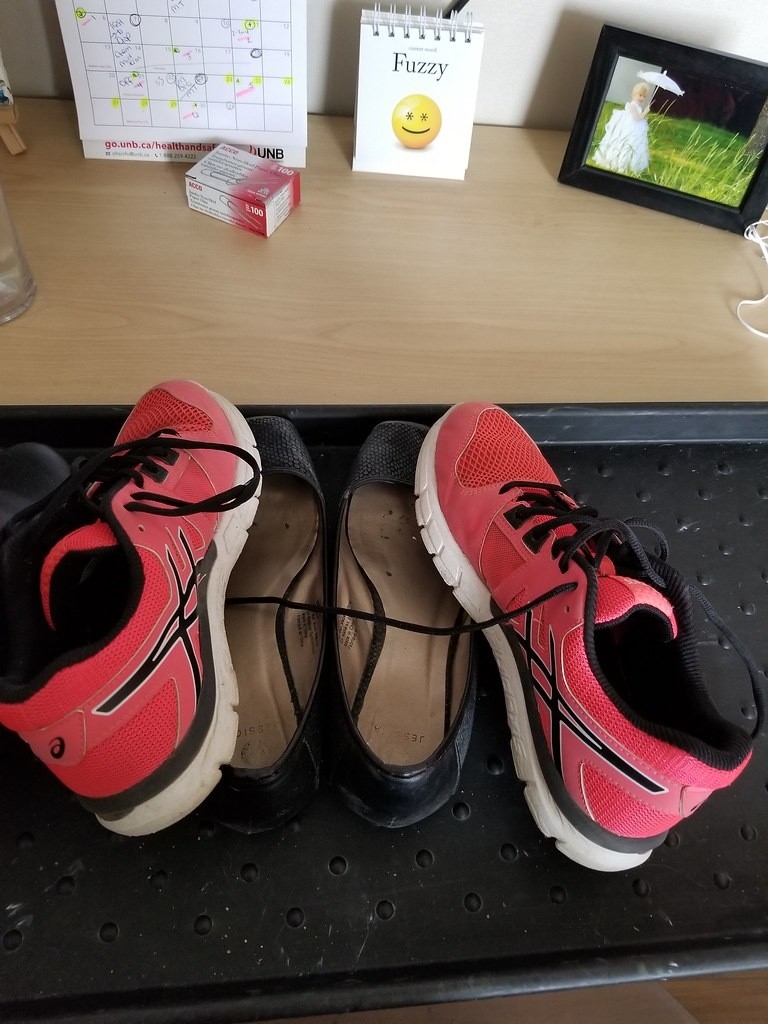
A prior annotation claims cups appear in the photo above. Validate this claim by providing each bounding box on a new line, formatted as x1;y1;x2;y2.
0;189;37;326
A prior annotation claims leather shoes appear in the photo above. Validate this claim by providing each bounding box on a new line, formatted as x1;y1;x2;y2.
198;414;482;830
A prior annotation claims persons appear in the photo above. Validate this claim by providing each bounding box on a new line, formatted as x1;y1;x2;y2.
592;83;650;173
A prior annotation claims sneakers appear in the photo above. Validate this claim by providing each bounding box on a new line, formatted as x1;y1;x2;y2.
2;377;262;837
414;399;766;873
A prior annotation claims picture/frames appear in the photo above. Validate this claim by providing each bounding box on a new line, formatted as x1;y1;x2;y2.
557;23;768;238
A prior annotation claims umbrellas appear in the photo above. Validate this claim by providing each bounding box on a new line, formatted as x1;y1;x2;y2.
637;70;685;104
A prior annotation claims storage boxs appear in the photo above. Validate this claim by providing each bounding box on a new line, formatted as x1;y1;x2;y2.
184;142;301;239
